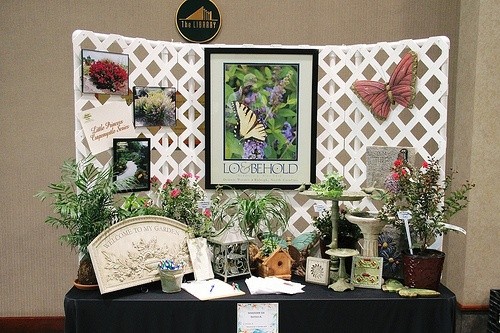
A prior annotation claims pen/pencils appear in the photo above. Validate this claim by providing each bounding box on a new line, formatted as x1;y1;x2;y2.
157;258;186;270
210;285;214;292
232;282;240;290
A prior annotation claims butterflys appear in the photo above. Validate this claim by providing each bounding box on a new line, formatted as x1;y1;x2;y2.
232;101;269;144
352;50;417;121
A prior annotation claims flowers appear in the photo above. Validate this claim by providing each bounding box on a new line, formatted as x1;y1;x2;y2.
373;156;476;254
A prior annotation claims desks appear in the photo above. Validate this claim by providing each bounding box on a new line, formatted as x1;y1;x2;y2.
63;269;457;333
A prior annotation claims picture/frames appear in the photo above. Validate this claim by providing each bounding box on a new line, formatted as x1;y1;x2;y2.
81;48;129;95
113;138;151;193
204;47;319;190
350;256;383;289
133;86;176;127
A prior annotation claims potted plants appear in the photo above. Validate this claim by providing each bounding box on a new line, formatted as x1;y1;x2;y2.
403;249;446;289
311;209;364;275
33;152;124;291
